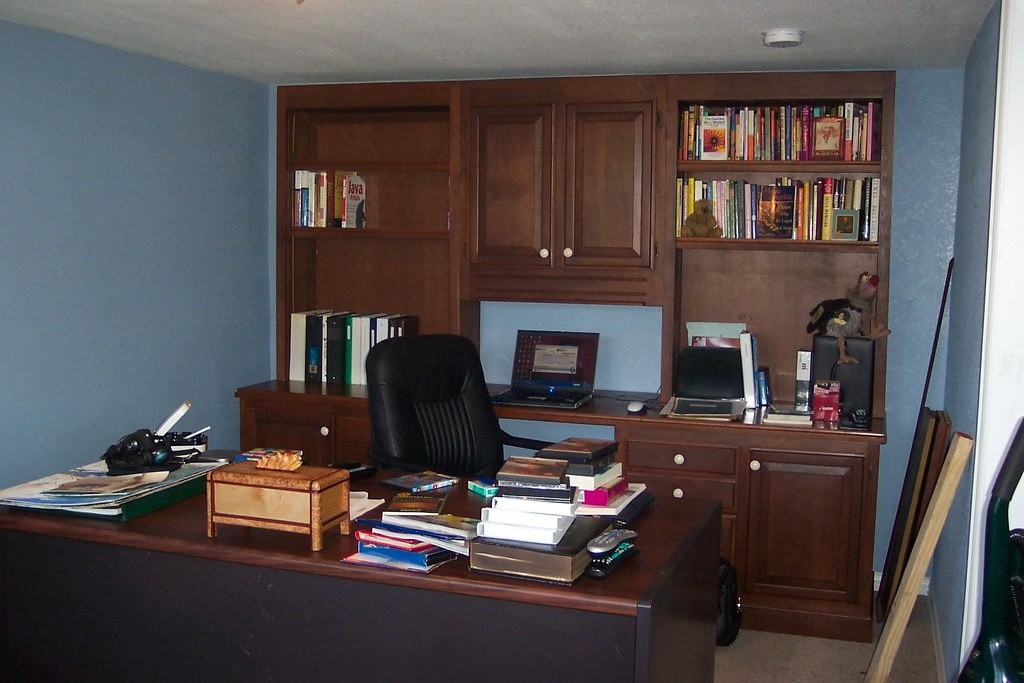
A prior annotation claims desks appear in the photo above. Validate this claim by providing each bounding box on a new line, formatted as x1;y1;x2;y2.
0;447;722;683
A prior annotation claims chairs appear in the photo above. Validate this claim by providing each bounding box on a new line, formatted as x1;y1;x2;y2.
364;334;555;477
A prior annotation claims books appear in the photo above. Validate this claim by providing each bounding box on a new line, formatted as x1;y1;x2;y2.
0;104;882;587
497;456;569;485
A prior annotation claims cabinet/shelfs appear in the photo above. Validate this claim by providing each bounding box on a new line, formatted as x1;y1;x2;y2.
232;70;896;643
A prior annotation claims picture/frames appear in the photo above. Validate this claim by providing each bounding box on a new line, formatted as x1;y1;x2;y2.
830;208;861;241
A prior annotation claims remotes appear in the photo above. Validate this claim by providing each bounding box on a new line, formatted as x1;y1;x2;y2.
585;540;640;578
586;528;638;557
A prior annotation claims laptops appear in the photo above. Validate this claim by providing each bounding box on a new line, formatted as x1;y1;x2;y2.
491;329;599;409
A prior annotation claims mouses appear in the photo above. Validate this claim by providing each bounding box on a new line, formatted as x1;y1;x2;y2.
627;401;648;415
